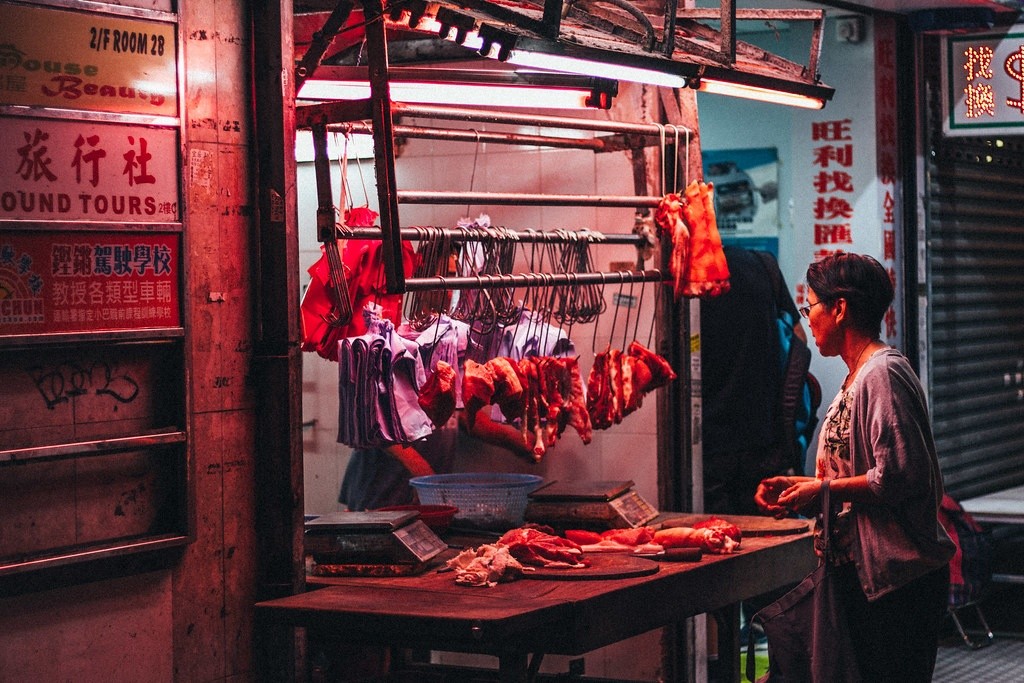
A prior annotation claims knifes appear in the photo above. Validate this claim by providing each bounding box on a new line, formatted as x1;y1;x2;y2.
585;548;702;560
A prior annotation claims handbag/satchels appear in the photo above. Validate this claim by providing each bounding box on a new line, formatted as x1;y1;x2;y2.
746;479;862;683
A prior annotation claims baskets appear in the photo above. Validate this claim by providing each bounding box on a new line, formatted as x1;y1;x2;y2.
409;472;542;532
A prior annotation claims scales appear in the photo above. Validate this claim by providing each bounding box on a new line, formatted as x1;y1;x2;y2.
524;479;661;531
302;510;450;567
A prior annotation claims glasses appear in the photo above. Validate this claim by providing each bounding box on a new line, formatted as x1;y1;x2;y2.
799;298;826;318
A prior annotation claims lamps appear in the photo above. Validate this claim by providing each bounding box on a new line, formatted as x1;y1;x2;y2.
384;1;836;110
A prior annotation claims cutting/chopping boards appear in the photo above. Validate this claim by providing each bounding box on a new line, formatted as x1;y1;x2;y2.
517;552;660;579
661;515;809;535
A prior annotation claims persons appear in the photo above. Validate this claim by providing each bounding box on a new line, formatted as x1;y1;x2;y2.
754;251;958;683
338;238;549;511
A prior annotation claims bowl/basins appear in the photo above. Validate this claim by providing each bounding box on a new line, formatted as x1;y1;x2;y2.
375;505;457;533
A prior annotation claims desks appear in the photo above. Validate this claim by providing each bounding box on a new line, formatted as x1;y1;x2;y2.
253;513;818;683
959;485;1024;641
